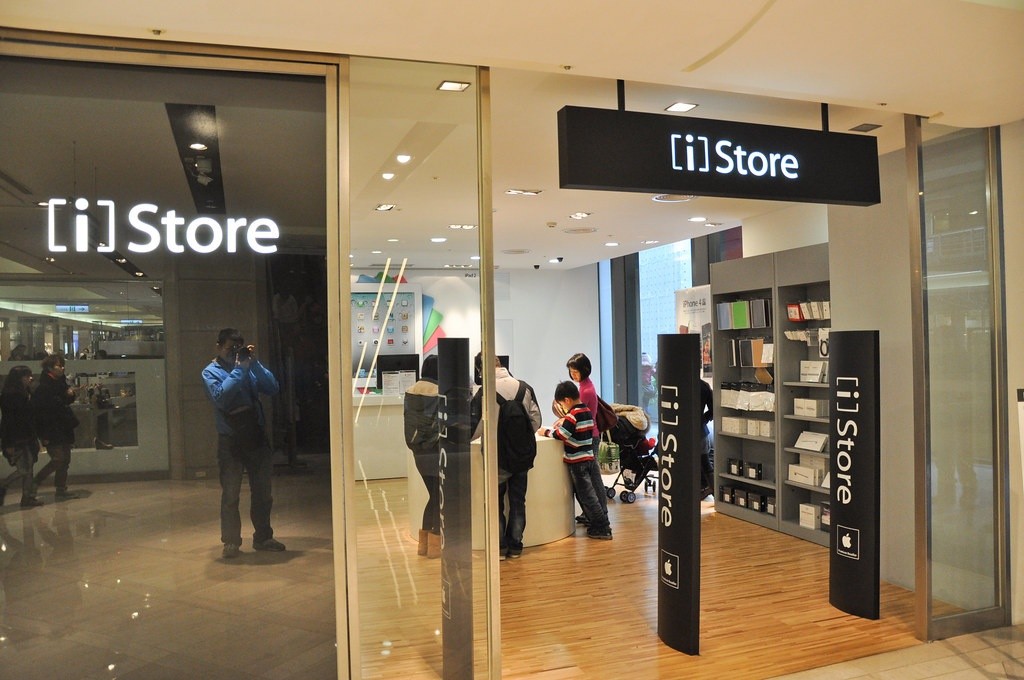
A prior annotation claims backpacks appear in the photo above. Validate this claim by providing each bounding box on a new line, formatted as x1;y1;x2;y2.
596;394;617;432
496;379;538;475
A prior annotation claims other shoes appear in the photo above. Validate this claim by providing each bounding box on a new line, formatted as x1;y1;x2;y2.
54;486;80;500
20;495;40;507
587;526;612;539
584;519;592;526
575;515;585;521
0;477;8;506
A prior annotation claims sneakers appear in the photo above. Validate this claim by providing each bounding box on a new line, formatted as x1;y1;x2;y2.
500;541;523;560
222;543;239;558
252;533;285;552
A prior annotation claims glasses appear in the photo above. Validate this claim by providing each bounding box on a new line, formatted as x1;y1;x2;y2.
56;364;64;367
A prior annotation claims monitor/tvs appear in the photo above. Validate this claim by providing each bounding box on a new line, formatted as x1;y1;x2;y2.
377;354;419;389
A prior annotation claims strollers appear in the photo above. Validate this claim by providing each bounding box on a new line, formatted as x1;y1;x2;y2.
604;404;660;506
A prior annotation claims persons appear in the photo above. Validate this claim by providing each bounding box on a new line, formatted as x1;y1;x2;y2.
403;354;442;559
0;342;107;508
201;328;286;559
553;353;608;526
469;354;542;561
538;381;613;539
639;433;655;455
700;379;716;497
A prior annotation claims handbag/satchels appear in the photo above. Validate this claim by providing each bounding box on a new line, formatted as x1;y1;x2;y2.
217;400;260;435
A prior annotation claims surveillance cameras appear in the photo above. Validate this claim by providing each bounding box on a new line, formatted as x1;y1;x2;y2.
534;265;539;269
557;258;563;262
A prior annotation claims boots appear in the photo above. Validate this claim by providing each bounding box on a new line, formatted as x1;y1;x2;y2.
427;531;441;559
417;528;432;555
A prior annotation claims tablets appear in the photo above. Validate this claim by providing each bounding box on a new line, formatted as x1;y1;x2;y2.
351;282;423;388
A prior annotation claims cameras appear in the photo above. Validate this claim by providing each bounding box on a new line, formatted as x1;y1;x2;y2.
230;345;250;362
